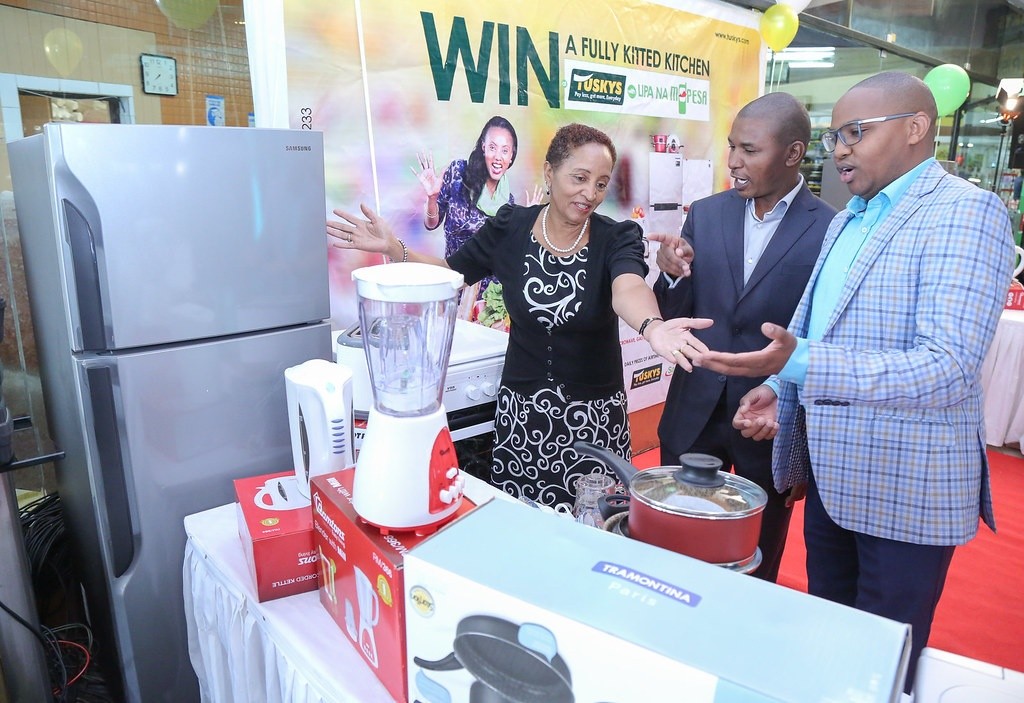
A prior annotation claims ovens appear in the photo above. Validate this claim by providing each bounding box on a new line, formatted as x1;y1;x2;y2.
445;401;498;482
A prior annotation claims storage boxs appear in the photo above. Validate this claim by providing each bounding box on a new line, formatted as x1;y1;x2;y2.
310;468;477;703
233;471;319;604
1004;283;1024;310
403;496;912;703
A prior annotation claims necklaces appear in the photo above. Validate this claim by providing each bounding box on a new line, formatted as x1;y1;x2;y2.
542;203;588;253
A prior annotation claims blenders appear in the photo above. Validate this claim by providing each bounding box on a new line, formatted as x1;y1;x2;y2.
352;262;466;536
354;565;378;669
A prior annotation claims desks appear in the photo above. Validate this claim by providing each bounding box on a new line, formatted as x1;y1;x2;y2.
183;469;531;703
980;309;1024;456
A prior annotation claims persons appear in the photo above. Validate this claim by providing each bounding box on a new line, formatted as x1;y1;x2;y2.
411;116;544;309
328;123;714;513
646;91;839;584
699;72;1016;696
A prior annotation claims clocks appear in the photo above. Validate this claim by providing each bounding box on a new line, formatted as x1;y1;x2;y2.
140;53;178;96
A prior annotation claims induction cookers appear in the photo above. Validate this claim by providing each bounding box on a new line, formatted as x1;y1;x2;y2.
332;318;509;417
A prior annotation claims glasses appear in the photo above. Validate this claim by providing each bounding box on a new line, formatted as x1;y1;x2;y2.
821;114;920;153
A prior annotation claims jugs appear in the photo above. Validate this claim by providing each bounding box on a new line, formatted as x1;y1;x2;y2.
570;476;627;533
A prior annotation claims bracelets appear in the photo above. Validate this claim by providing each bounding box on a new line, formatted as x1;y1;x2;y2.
426;203;439;218
638;318;664;336
389;237;407;263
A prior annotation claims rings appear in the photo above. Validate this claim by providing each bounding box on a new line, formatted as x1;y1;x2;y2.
681;346;684;353
672;350;679;356
348;234;352;242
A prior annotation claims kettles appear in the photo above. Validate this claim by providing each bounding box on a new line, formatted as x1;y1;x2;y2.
284;358;357;499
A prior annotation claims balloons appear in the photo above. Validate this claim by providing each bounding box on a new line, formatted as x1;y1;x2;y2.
760;3;799;52
923;64;970;117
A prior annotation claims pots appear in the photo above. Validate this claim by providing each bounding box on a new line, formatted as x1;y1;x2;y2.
412;615;577;703
650;133;684;154
573;441;768;564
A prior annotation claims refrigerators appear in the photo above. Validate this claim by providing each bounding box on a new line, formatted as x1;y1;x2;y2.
648;151;684;240
5;122;331;702
